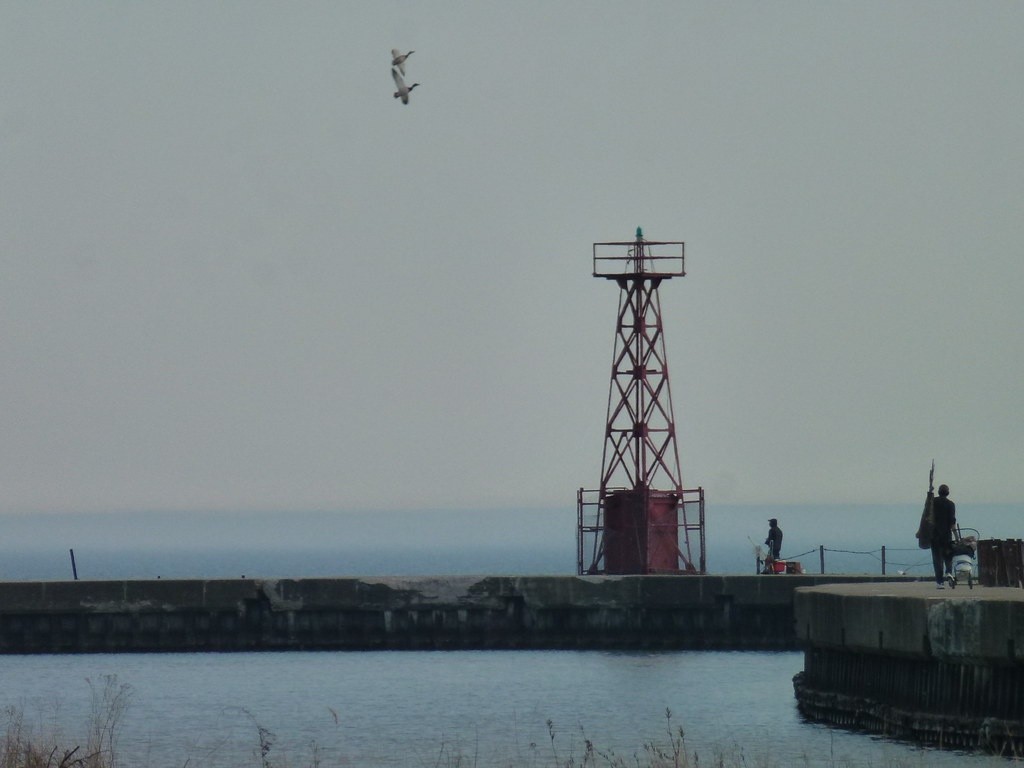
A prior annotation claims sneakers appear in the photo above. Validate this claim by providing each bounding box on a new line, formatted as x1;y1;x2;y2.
945;571;956;586
937;583;945;589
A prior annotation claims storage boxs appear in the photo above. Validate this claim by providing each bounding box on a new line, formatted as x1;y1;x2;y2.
786;561;801;574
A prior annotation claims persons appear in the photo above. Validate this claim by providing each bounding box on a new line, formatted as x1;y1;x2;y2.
760;518;783;575
915;484;961;589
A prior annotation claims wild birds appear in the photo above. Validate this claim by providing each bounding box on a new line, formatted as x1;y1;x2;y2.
386;46;416;77
386;66;421;106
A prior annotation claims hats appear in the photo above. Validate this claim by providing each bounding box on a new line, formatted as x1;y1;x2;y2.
768;518;777;522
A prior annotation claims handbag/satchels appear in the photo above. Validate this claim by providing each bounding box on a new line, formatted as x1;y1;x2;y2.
918;492;936;549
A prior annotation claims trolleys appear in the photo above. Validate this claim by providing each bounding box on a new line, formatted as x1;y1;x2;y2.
939;524;981;589
